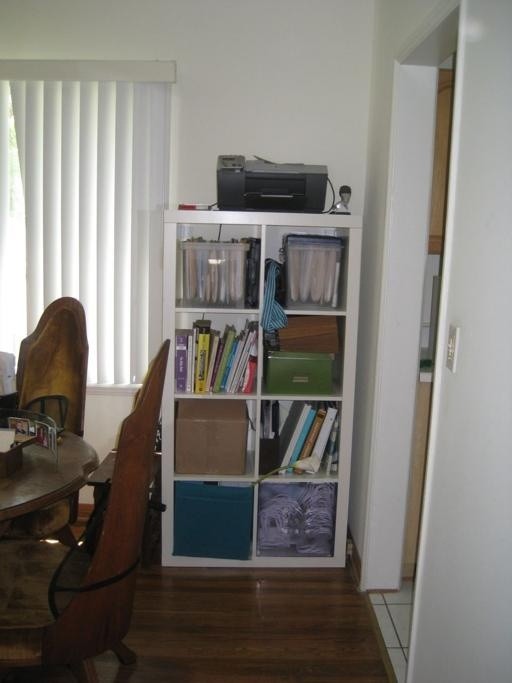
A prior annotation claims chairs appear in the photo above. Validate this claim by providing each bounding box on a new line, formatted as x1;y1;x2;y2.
2;339;170;682
16;298;91;536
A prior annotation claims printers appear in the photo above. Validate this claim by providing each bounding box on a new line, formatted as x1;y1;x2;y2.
217;154;328;214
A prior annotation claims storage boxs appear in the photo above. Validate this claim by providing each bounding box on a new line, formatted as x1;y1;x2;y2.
264;351;337;394
282;234;342;312
184;241;249;310
170;479;255;561
174;398;256;476
276;315;340;353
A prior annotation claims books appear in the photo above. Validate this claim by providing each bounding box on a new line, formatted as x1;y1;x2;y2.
283;231;341;310
271;400;341;476
174;232;259;397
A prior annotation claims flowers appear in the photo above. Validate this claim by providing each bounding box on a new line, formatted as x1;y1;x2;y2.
254;452;320;484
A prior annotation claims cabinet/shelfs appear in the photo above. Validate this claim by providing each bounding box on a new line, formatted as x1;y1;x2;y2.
159;205;364;568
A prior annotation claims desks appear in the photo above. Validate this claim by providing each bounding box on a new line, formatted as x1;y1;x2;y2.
3;426;94;538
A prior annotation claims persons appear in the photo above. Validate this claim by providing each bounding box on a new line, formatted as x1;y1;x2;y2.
15;421;26;434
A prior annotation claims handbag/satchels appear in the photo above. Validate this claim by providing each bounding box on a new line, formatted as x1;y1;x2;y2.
265;258;285;306
